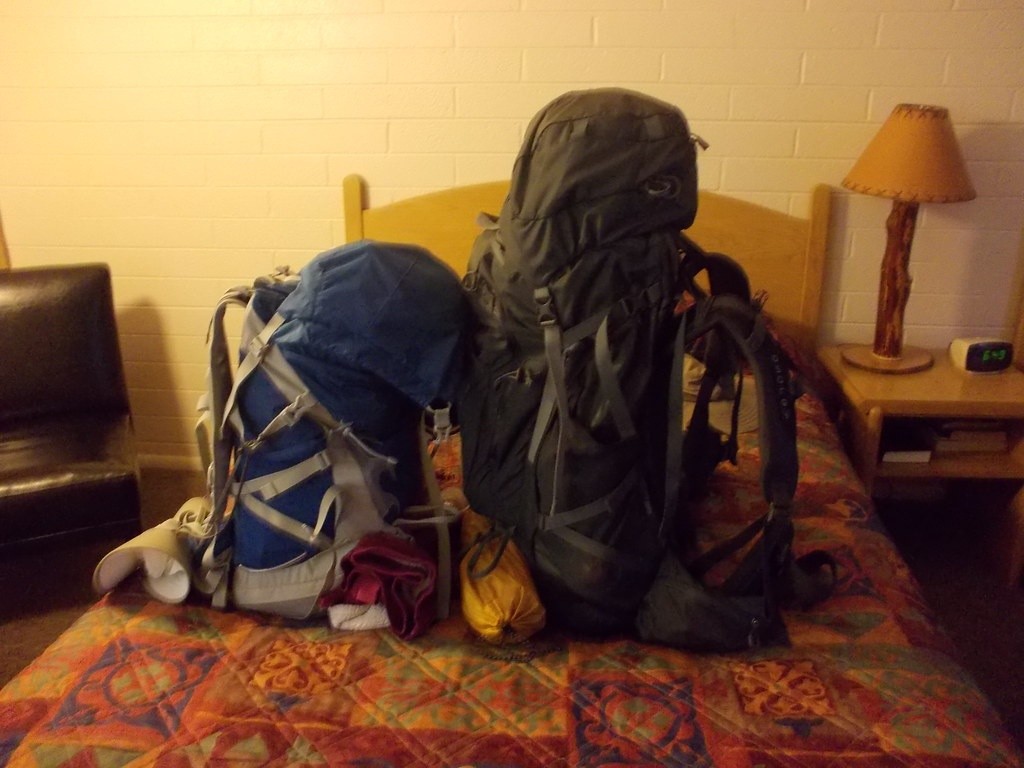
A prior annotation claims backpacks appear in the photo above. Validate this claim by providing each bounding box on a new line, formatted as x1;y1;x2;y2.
194;239;461;632
460;86;837;650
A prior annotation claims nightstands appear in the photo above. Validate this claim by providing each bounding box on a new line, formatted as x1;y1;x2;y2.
814;341;1024;596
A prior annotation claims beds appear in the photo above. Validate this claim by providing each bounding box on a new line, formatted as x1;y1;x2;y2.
0;87;1024;768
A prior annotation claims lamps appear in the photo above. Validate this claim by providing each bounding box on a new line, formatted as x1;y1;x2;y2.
840;102;979;375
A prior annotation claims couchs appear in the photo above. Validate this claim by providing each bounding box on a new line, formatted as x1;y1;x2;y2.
0;263;148;623
